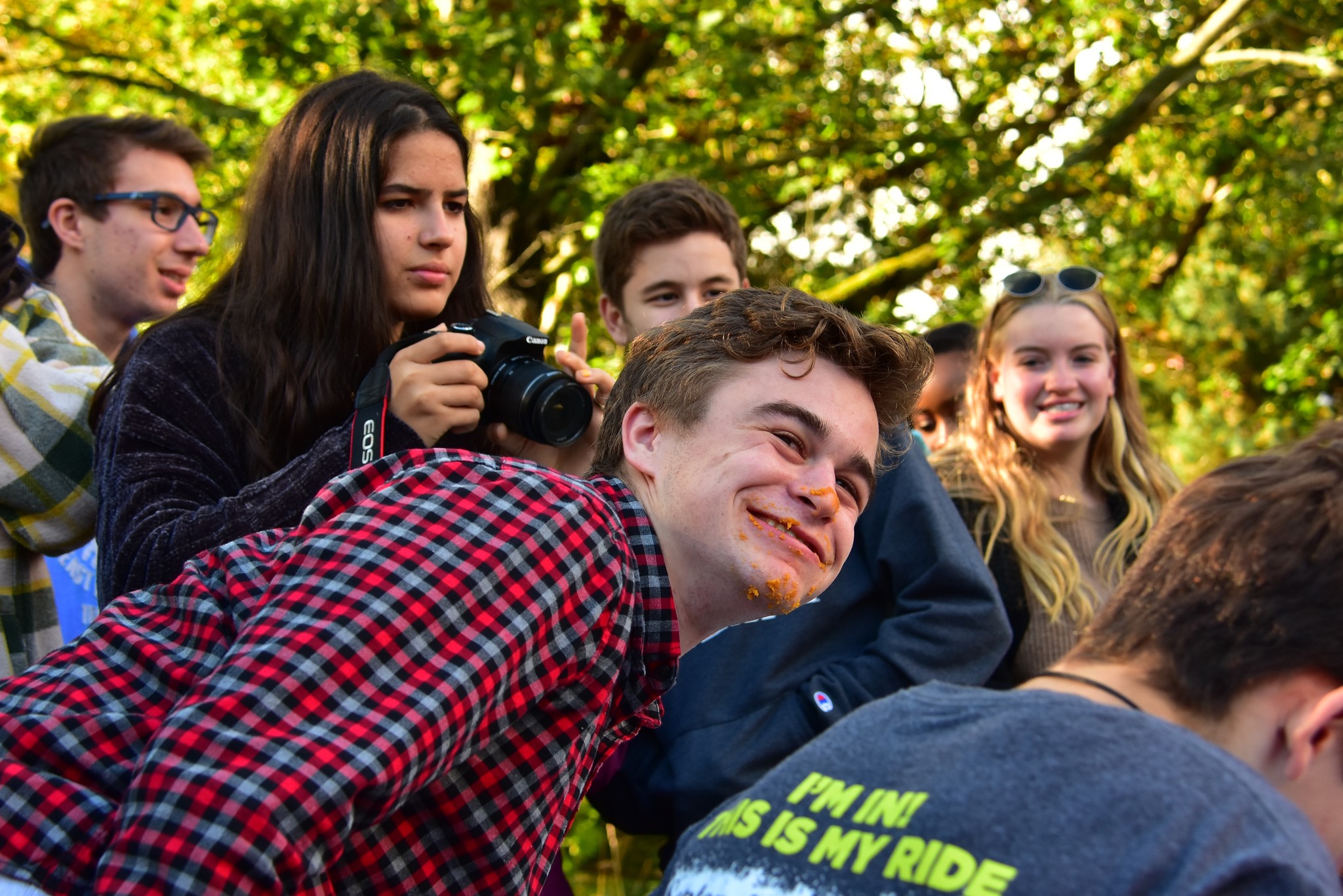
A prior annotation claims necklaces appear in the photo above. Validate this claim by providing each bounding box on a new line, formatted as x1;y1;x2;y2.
1037;670;1140;709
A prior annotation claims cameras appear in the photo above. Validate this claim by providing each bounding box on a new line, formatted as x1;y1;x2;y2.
430;309;591;449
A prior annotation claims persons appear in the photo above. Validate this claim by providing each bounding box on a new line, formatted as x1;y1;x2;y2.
0;284;930;896
662;408;1343;896
0;113;219;687
910;316;988;454
926;266;1183;692
99;69;610;618
538;175;1016;835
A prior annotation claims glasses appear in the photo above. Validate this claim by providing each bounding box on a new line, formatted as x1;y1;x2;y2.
40;191;218;248
991;265;1104;326
